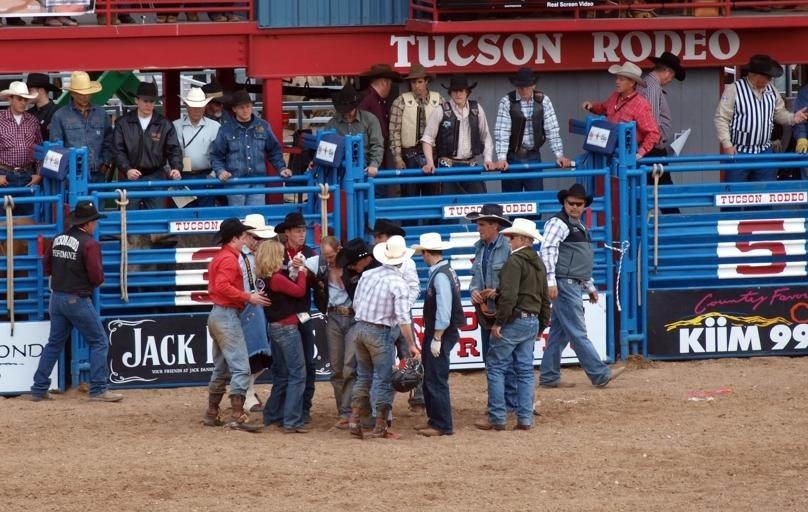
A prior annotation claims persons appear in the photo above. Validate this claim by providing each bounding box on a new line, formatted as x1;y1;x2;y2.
636;52;686;213
466;202;548;429
582;61;661;160
30;201;124;403
540;183;626;387
1;0;240;27
793;84;808;180
207;210;466;439
0;65;569;320
713;55;808;211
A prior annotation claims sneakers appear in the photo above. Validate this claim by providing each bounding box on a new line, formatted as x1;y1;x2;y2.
0;12;241;26
30;393;54;401
89;391;123;402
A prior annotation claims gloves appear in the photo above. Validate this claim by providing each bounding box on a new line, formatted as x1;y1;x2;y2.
431;337;441;358
795;138;808;153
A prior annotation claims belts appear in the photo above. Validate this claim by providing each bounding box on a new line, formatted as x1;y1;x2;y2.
0;163;34;176
451;157;474;163
358;320;391;329
328;305;354;316
517;311;536;316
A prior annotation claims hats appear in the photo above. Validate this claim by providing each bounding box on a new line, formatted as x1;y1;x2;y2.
68;201;107;225
